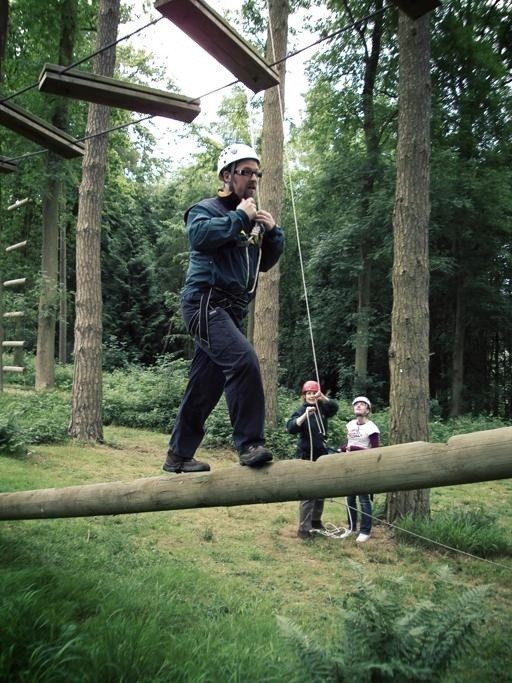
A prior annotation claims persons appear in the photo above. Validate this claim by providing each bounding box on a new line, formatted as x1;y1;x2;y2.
159;142;286;475
339;396;380;543
286;380;339;541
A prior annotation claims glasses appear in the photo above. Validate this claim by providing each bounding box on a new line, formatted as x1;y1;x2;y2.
233;169;263;178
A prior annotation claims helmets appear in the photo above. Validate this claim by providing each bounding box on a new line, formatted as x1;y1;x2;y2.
217;143;260;182
302;380;319;393
352;396;371;408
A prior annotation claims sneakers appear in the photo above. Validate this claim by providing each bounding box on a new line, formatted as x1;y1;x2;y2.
239;445;273;466
297;529;315;540
340;528;357;539
312;520;326;532
355;532;371;544
163;449;210;473
